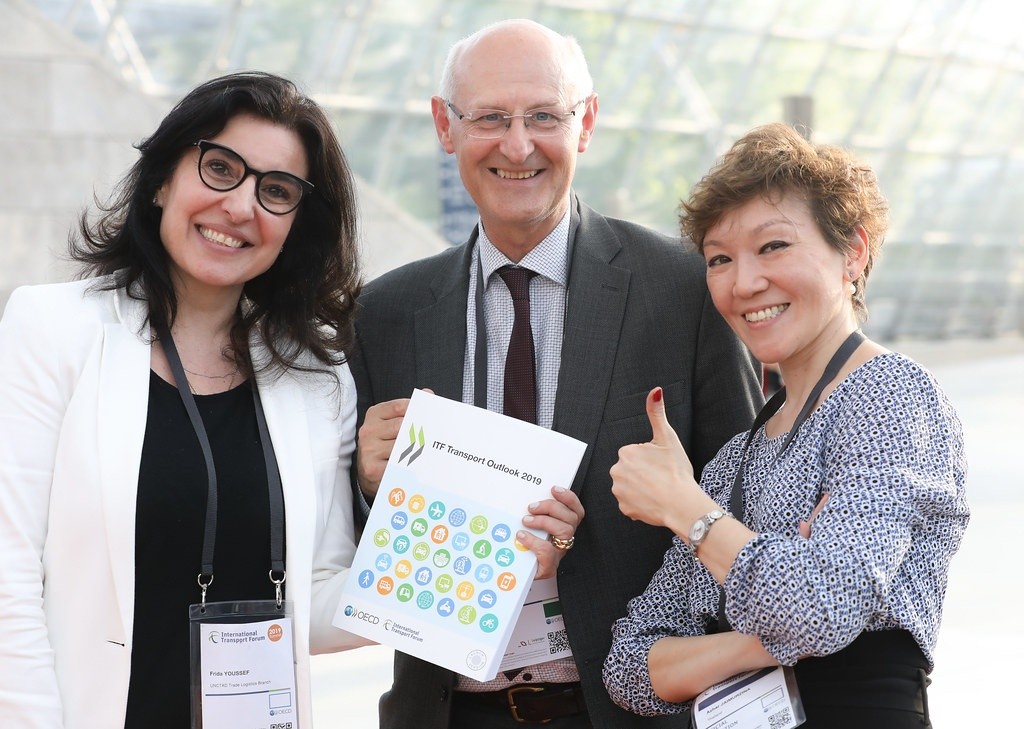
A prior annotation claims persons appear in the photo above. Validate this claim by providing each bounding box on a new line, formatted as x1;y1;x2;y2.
335;19;768;729
2;71;587;729
599;122;970;729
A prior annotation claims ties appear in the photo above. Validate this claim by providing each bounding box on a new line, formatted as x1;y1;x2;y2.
493;265;541;682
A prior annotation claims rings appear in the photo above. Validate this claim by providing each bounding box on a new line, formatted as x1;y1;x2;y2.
551;534;575;551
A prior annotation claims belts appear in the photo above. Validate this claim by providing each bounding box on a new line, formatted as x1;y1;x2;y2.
451;683;587;724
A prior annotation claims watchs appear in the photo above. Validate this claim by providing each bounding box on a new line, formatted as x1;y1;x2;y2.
689;510;735;557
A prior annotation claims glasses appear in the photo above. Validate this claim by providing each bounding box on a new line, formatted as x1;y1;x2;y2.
446;97;587;140
192;138;315;215
